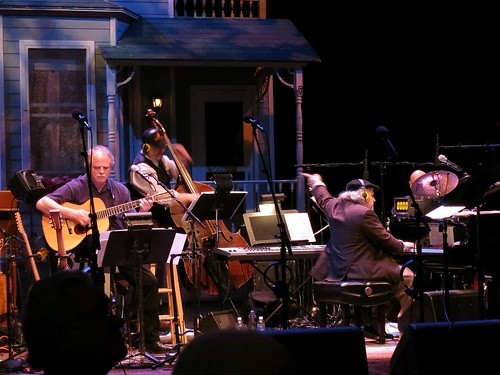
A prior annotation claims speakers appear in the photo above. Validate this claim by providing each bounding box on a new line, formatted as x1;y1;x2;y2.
397;289;481;332
388;319;500;375
171;325;369;375
195;309;239;336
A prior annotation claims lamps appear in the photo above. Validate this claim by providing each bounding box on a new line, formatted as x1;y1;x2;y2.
152;94;164;113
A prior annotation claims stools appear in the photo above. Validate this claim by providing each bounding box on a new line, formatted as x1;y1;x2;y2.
314;280;394;344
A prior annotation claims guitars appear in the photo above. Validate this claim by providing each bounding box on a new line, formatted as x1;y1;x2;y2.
47;209;74;272
41;189;176;253
14;210;41;281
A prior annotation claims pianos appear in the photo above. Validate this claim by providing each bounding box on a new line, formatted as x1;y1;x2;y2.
390;209;500;341
217;243;327;327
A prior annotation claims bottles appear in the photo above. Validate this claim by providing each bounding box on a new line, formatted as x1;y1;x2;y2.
236;317;244;331
248;310;256;330
257;316;266;331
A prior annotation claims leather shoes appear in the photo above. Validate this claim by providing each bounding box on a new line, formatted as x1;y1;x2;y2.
144;340;175;353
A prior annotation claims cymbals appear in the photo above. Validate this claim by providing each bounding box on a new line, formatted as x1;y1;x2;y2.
483;181;500;197
411;169;459;199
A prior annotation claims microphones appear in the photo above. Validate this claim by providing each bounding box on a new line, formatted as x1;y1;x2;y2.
481;182;500;198
240;114;266;132
438;155;472;180
376;126;395;149
72;108;92;130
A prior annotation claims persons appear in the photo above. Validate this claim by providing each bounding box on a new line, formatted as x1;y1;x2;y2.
36;146;175;354
128;127;201;233
391;170;438;241
300;173;415;320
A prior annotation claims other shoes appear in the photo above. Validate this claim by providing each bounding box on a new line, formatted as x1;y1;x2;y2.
363;326;393;341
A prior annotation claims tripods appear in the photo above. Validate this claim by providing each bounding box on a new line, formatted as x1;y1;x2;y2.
102;230;177;368
152;253;189;369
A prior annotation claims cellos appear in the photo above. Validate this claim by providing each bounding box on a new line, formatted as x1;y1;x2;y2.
144;107;255;319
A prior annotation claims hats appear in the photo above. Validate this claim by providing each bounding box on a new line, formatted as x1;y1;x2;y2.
346;179;379;191
143;128;168;149
